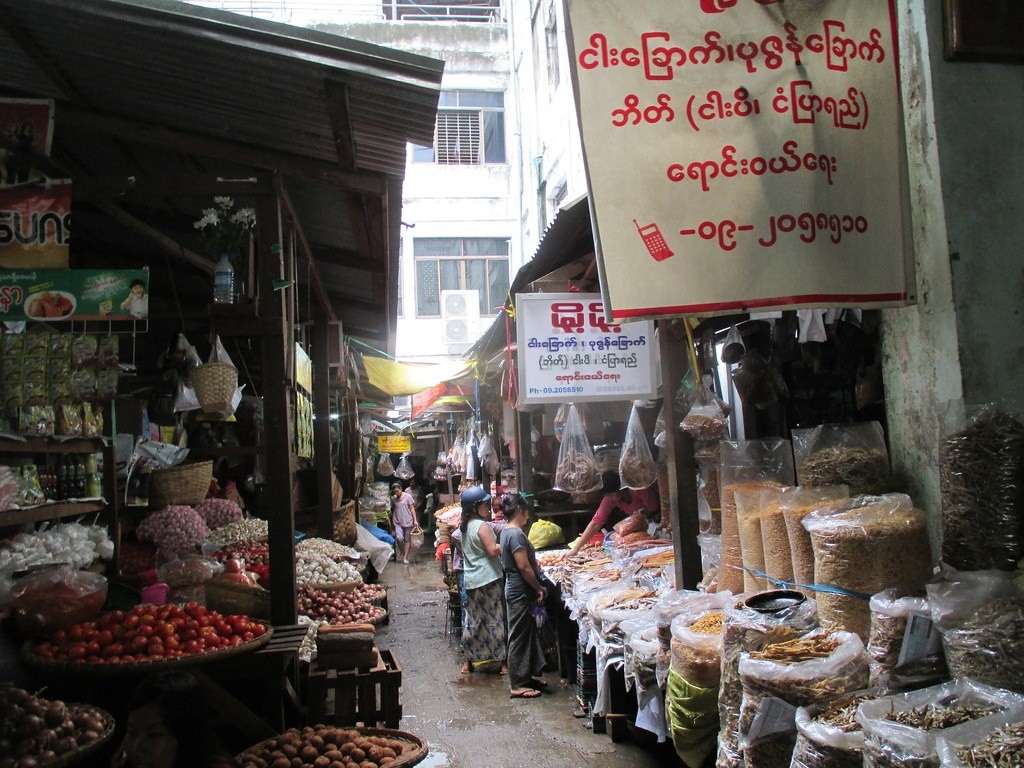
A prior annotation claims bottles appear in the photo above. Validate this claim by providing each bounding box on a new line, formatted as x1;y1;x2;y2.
39;453;101;500
213;253;234;305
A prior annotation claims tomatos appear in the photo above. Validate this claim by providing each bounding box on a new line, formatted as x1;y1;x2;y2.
30;601;266;664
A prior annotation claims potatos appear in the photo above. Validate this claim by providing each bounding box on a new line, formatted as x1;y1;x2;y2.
241;724;402;768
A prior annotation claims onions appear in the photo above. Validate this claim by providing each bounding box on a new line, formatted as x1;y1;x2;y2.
0;683;106;768
296;581;383;625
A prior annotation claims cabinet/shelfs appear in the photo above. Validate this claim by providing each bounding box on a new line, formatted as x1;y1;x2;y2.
445;600;461;648
0;436;121;627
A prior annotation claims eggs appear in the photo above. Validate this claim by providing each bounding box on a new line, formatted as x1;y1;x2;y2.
539;554;566;567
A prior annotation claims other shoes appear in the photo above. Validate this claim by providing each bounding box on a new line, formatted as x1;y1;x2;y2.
398;554;403;560
404;560;409;564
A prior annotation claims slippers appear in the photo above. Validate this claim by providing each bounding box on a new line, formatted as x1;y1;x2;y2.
462;664;475;673
510;688;541;698
500;668;509;674
522;679;547;687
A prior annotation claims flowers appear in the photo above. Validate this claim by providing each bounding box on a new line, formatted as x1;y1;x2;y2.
192;196;256;252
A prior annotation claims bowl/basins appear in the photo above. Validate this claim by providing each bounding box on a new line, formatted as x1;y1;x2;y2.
743;589;807;615
24;290;75;320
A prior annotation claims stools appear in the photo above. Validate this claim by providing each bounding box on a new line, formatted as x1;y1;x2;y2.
369;582;389;625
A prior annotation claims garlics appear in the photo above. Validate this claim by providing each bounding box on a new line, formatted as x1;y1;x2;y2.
295;549;359;585
135;498;244;559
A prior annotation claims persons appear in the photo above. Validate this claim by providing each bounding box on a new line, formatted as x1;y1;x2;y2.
405;478;424;526
458;487;508;674
451;522;510;645
498;493;550;698
391;481;418;564
562;470;661;557
120;279;148;319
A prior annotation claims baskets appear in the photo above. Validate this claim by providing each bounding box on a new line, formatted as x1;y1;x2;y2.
139;460;212;510
190;365;238;414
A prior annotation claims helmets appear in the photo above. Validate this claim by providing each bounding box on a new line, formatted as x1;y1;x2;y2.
461;487;491;506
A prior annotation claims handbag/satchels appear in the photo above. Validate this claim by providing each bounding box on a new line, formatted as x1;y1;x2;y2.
410;525;424;548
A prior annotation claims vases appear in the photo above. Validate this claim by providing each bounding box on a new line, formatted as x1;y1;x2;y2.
213;253;234;304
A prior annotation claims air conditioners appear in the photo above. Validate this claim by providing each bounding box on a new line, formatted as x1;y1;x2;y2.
439;289;480;345
